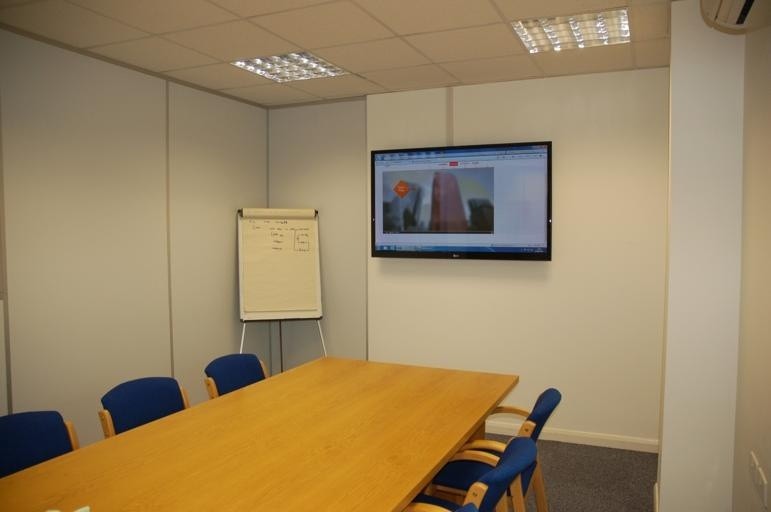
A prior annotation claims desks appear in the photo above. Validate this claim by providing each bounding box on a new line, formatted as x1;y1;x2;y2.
0;355;520;512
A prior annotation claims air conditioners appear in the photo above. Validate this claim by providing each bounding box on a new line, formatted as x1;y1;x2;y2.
699;0;771;35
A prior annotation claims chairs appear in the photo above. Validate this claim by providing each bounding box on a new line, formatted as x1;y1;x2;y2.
0;409;79;479
461;387;561;512
202;353;269;400
401;503;479;512
96;377;190;439
424;437;537;512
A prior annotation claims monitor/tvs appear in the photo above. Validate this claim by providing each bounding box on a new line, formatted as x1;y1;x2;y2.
370;140;553;262
